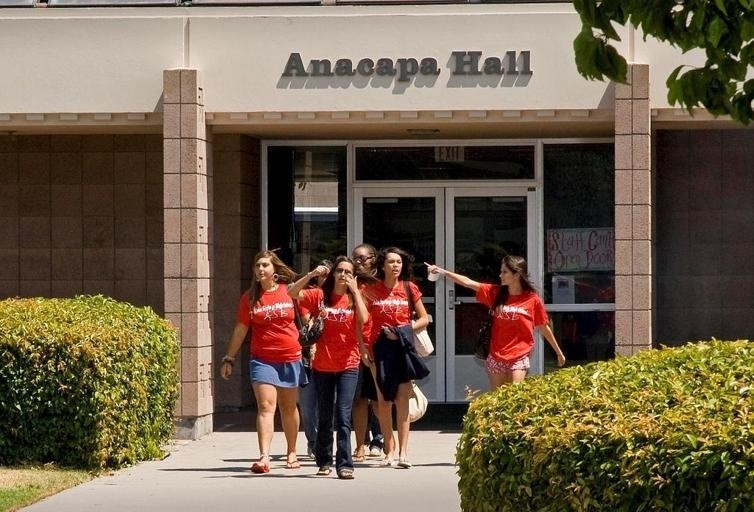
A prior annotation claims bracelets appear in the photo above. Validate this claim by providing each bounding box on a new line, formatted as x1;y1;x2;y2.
223;356;235;367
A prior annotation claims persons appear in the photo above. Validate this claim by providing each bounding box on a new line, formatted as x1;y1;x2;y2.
221;252;317;472
287;244;430;478
551;268;615;360
423;253;567;393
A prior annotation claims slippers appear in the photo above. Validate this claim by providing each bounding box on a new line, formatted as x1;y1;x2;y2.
308;447;332;475
251;462;269;473
337;469;353;479
352;443;381;462
287;461;300;468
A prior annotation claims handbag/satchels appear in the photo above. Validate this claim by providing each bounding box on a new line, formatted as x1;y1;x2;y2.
412;311;435;358
298;317;324;360
409;379;428;422
473;315;491;359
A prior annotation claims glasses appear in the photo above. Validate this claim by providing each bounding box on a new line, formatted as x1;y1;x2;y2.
351;255;373;263
335;268;351;274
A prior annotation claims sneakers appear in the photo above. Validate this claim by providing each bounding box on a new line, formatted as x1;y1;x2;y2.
398;452;412;467
380;454;393;467
